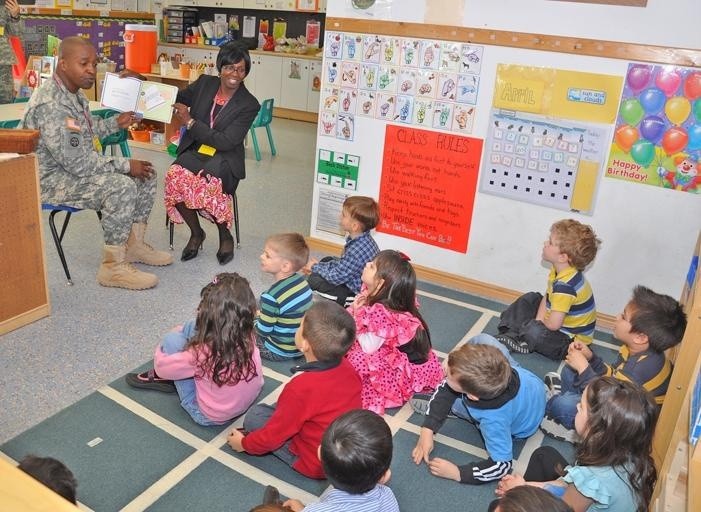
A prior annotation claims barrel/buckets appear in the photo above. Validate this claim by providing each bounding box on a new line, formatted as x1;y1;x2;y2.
122;23;159;74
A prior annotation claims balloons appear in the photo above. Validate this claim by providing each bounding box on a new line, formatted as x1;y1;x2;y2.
614;65;701;168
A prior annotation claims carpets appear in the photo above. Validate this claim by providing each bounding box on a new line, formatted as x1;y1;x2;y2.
1;278;625;512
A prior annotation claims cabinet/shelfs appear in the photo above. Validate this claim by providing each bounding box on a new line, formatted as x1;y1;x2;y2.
647;233;699;512
156;1;327;123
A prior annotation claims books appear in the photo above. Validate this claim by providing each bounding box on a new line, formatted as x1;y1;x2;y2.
14;34;64;101
98;71;179;125
187;20;231;39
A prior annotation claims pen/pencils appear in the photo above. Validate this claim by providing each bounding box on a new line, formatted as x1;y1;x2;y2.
162;54;170;62
187;62;206;71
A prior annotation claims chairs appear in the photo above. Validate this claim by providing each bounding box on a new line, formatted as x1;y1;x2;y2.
0;109;130;159
41;202;103;286
166;189;240;249
242;99;276;161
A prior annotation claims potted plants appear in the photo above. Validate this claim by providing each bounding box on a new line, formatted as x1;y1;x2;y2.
149;128;166;145
125;121;154;141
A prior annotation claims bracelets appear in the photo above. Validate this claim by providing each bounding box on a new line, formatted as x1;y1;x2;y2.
186;116;195;132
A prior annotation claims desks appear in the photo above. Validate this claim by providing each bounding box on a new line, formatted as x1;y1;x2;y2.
0;151;51;335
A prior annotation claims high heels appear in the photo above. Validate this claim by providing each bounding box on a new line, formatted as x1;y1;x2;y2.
216;235;234;265
181;229;206;261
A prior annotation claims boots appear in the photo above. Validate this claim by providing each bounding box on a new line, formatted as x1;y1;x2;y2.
96;222;174;290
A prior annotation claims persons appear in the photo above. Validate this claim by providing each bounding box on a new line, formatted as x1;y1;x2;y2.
15;34;175;289
299;195;381;311
125;271;266;428
227;298;365;481
411;332;551;486
492;218;604;366
164;40;261;265
17;453;78;508
290;248;446;417
250;409;403;512
487;376;658;512
0;0;23;103
538;285;688;448
250;230;317;364
493;486;575;512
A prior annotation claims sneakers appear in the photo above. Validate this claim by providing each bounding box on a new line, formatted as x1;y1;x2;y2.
409;395;458;419
539;411;584;446
493;332;529;354
125;368;175;393
543;372;562;402
